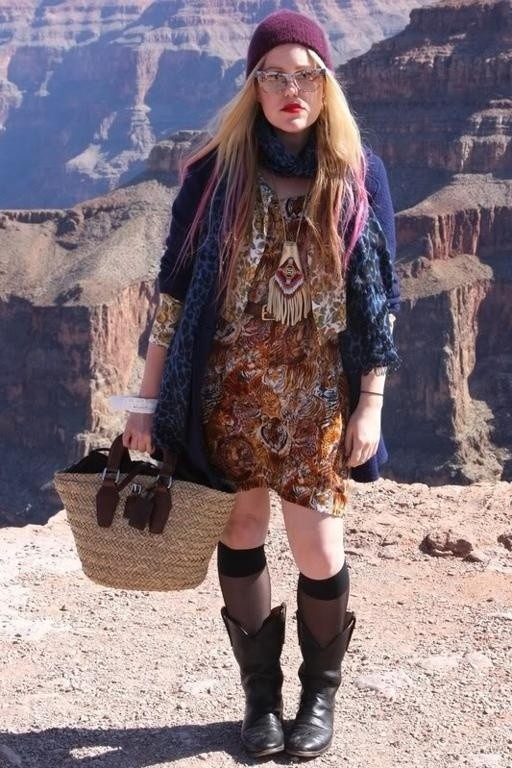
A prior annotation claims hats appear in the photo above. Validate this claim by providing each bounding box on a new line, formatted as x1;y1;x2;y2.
246;9;333;78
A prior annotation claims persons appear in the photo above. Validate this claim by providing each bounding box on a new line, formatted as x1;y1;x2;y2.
121;10;401;759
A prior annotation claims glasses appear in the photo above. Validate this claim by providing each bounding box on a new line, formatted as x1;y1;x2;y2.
254;66;327;94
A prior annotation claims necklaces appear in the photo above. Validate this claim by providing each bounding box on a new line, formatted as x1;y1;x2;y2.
266;171;313;328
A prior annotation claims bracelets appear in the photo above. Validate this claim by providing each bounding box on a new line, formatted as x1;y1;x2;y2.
360;390;386;397
126;396;159;414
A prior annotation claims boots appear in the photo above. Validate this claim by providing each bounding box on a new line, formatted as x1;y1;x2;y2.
286;608;357;758
221;605;285;758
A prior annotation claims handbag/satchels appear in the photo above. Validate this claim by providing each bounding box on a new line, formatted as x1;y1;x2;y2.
54;434;240;593
